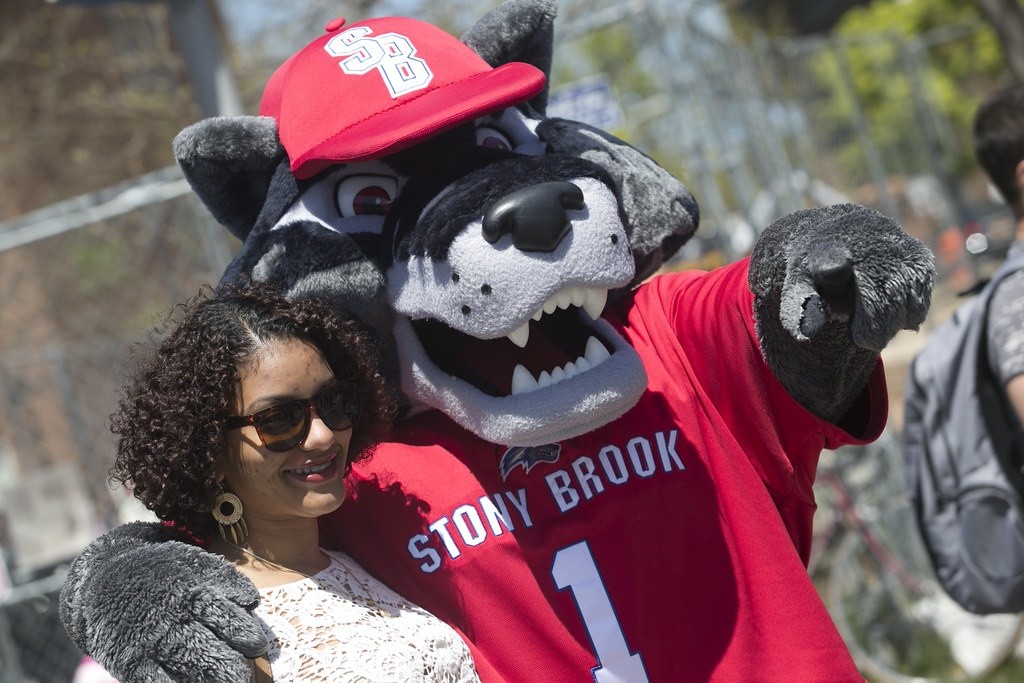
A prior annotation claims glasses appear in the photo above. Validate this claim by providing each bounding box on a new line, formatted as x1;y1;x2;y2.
221;379;364;452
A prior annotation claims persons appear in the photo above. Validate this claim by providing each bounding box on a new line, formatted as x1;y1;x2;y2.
972;81;1024;429
70;288;481;683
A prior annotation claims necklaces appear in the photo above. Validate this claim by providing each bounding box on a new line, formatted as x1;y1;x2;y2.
224;539;390;617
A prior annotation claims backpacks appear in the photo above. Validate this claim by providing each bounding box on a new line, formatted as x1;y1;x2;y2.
902;264;1024;616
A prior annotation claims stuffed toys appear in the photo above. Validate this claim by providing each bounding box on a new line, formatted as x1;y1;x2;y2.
57;0;934;682
